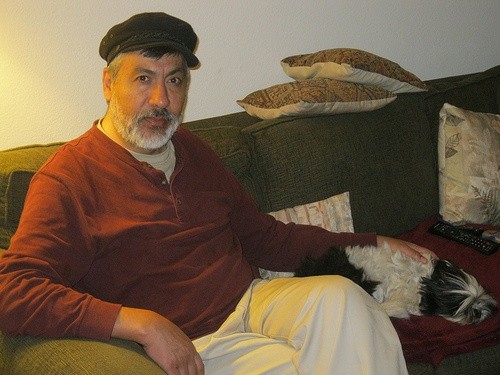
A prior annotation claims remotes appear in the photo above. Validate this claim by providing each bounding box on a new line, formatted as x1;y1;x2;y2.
482;230;500;244
454;225;484;236
429;221;499;255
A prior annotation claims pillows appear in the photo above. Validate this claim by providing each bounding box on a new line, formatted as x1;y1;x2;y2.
280;48;429;93
438;102;500;225
258;191;355;280
236;79;397;119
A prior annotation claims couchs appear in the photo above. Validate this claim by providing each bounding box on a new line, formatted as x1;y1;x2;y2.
0;66;500;375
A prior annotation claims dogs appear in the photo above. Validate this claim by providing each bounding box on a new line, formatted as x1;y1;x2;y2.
292;241;499;326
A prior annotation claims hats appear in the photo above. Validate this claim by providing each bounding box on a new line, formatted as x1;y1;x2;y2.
99;12;199;67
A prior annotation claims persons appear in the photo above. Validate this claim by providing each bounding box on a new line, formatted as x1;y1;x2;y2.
0;13;441;375
